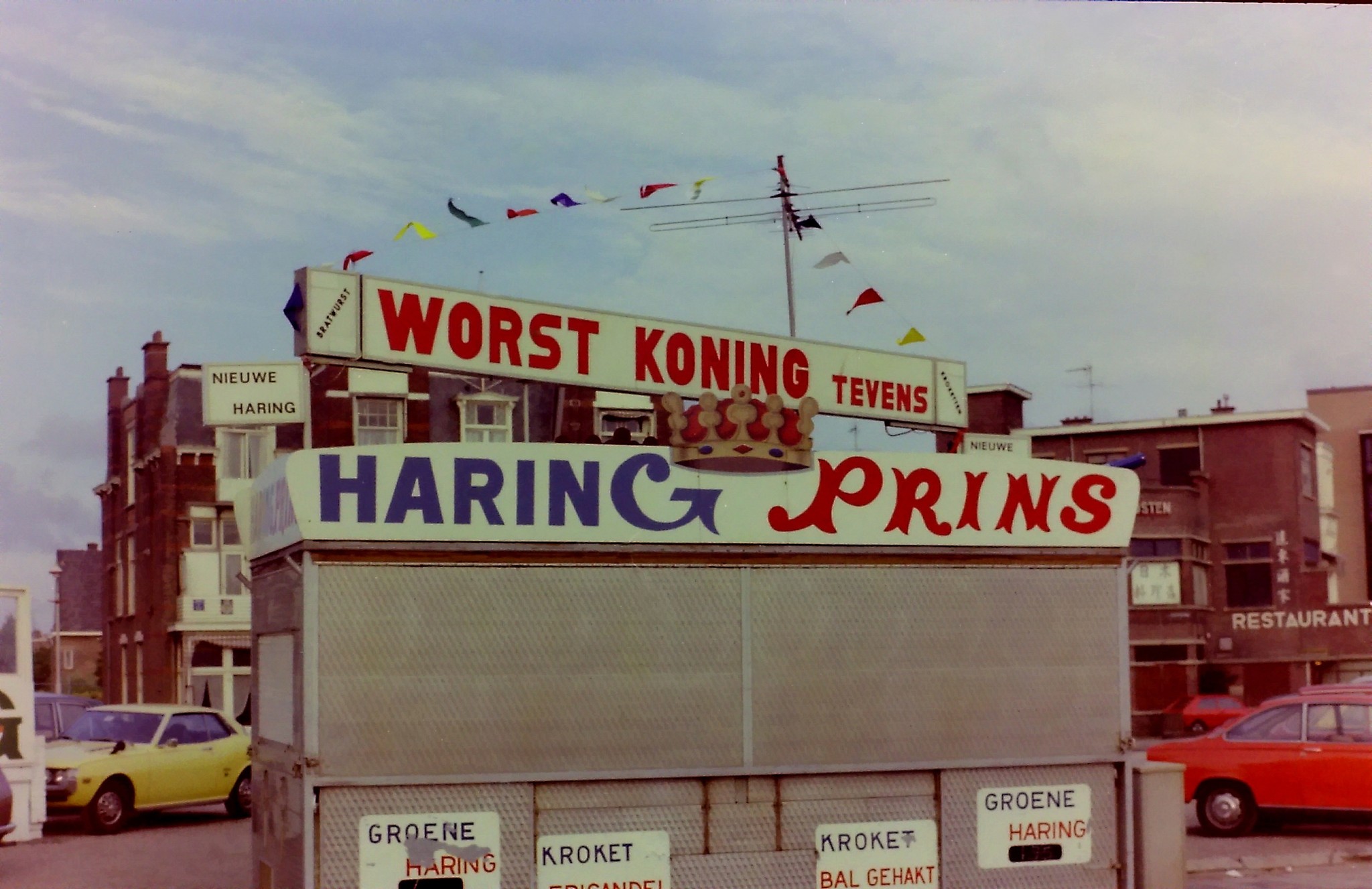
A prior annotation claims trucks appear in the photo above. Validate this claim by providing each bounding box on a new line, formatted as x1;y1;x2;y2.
0;584;48;846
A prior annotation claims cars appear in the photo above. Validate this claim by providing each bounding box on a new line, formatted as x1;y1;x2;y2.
1158;693;1252;739
32;691;105;742
43;703;255;834
1141;682;1372;837
